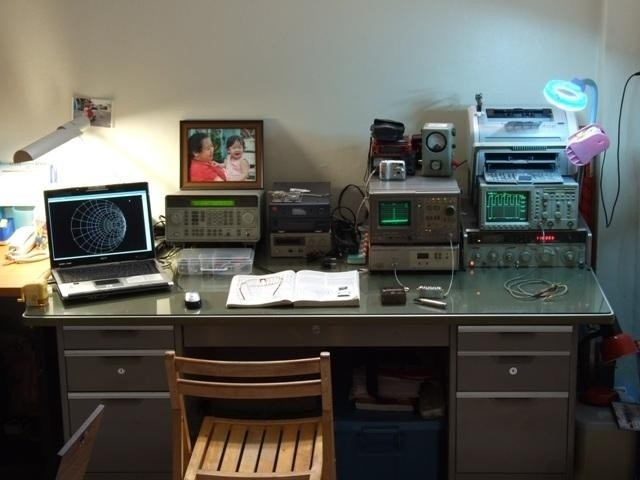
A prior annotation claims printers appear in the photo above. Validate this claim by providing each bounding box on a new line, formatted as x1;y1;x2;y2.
466;103;580;184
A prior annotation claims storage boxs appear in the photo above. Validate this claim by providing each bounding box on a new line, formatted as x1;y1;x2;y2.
575;404;640;480
334;401;441;480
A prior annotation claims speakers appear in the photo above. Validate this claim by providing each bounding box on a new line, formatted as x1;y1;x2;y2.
421;123;454;178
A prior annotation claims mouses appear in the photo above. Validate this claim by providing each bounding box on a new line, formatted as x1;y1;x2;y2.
184;291;201;309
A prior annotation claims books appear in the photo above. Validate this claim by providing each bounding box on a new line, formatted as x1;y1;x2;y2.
226;270;361;308
348;368;444;419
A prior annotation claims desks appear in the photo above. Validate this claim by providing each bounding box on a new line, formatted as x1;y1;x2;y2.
22;258;616;480
0;245;54;480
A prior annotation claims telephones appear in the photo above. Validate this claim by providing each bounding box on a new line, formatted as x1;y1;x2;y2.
6;225;38;256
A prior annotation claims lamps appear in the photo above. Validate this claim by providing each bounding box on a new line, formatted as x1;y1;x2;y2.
0;116;90;185
543;75;610;170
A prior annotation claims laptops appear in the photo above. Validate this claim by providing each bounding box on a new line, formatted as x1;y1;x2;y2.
43;181;174;305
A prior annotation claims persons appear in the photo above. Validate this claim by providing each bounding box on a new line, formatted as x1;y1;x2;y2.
188;133;250;181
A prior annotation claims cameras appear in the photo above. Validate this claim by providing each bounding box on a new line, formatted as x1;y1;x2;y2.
380;160;406;181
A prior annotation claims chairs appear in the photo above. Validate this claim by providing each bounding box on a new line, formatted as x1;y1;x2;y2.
165;350;337;480
52;403;105;480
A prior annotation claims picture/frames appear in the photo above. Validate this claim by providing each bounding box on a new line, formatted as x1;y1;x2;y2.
180;120;264;189
